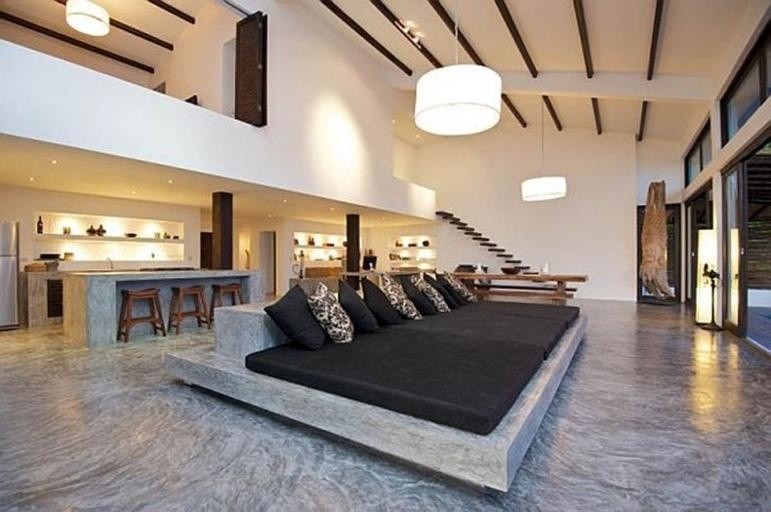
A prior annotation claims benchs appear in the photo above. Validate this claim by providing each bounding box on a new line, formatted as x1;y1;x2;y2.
472;289;574;306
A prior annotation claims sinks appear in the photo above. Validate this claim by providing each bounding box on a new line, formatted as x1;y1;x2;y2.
82;267;138;273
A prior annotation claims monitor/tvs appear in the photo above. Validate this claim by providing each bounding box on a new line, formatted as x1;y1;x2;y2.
363;256;376;274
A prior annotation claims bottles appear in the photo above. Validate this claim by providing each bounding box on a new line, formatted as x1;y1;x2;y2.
37;215;44;233
307;236;316;245
85;223;106;237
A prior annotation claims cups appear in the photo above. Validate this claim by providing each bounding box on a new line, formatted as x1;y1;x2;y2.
62;227;72;234
65;253;74;261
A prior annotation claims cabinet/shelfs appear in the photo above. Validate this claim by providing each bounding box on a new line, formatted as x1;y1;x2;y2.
294;244;347;266
385;246;437;264
32;210;186;262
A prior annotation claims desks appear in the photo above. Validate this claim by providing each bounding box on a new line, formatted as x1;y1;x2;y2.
61;269;265;349
450;272;588;306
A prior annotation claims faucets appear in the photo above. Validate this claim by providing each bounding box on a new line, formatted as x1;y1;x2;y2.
105;257;114;270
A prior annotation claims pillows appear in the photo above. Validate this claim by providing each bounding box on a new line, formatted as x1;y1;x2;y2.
263;269;480;351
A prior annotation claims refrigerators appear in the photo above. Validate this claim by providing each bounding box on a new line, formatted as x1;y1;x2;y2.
0;217;22;332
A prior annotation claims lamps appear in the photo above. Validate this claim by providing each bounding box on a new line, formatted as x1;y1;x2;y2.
413;0;503;135
66;0;111;36
520;100;567;202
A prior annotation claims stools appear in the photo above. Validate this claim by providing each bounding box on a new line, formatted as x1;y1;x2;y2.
210;284;245;322
168;285;211;335
117;288;167;342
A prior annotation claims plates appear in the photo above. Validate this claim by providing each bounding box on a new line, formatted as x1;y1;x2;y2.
123;233;139;238
